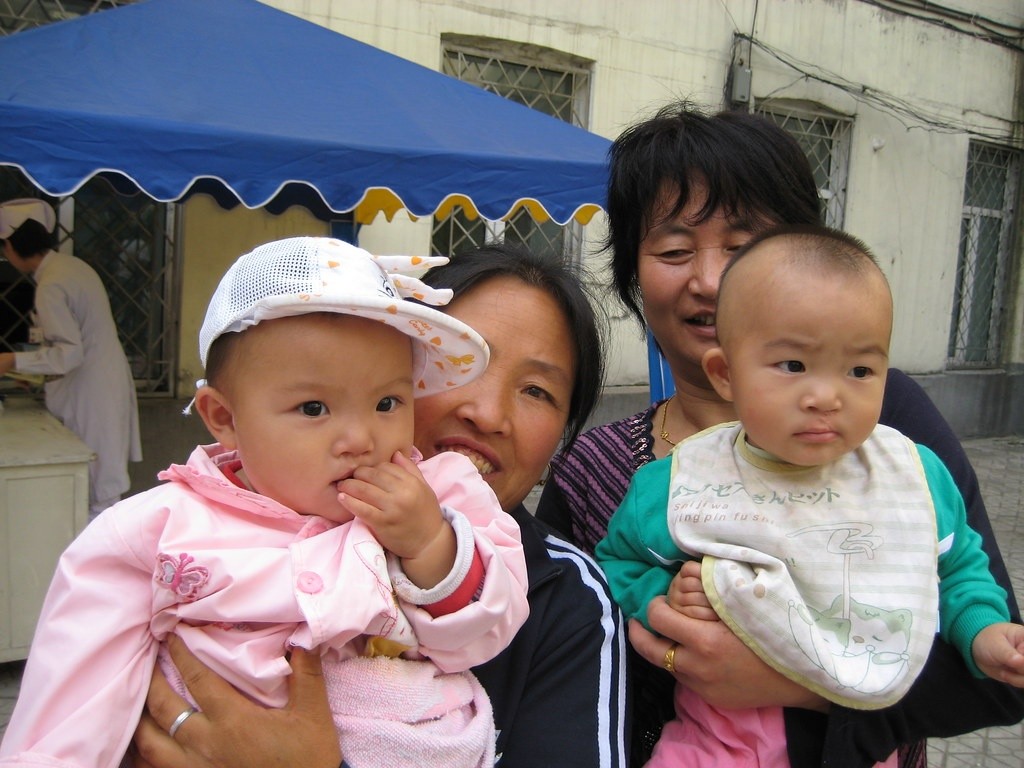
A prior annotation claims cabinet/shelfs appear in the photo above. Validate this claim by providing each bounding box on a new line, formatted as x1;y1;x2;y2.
0;397;97;665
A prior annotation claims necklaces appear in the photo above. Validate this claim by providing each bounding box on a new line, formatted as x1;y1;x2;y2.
660;394;676;446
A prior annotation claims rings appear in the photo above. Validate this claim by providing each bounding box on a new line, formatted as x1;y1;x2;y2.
664;643;680;673
168;708;197;737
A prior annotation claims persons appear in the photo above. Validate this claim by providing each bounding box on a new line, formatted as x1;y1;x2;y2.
531;99;1024;768
1;236;530;768
132;243;635;768
596;221;1024;768
0;196;145;513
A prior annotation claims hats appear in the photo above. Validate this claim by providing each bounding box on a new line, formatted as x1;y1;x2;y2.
183;234;491;399
0;198;56;239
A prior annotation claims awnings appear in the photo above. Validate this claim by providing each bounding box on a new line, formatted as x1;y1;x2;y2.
0;0;617;221
